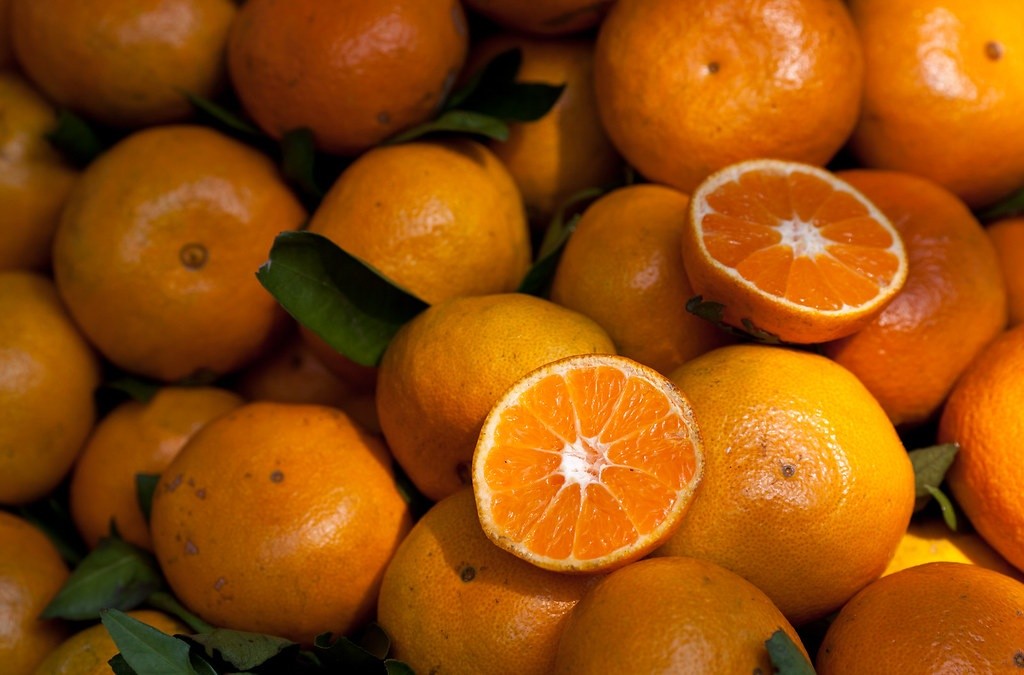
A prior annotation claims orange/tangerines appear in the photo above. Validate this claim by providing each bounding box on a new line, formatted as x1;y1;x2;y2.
0;0;1024;674
